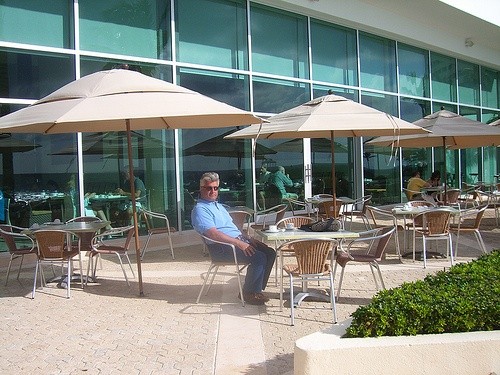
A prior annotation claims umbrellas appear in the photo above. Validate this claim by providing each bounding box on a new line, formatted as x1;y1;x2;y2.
344;135;420;174
0;133;41;154
183;127;276;177
47;129;183;190
267;137;349;173
221;88;432;220
0;62;269;297
364;105;500;205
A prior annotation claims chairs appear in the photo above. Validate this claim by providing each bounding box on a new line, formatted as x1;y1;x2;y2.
0;181;500;326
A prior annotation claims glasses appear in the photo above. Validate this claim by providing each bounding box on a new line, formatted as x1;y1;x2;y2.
201;186;219;191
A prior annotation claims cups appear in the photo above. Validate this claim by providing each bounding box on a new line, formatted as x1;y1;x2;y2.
287;223;294;230
54;219;60;224
269;225;277;232
403;202;411;210
32;223;39;229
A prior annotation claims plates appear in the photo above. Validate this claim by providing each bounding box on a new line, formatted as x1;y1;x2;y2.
265;230;279;232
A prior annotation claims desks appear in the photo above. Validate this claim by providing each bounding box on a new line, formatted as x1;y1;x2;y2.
304;197;356;203
89;195;132;241
192;190;233;203
20;221;111;289
251;225;360;308
394;206;461;261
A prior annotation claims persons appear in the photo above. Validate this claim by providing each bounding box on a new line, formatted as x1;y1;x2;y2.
327;171;340;193
426;170;442;202
266;165;298;210
191;172;276;306
257;161;269;209
114;163;148;237
65;167;114;230
406;170;432;201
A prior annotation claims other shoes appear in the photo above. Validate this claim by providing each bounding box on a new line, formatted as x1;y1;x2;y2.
258;293;271;302
237;291;265;306
